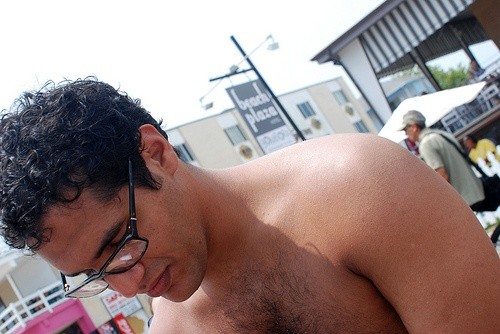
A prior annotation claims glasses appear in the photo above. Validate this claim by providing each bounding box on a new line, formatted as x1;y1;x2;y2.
60;153;149;298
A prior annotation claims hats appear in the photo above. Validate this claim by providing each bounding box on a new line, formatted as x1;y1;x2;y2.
397;110;425;131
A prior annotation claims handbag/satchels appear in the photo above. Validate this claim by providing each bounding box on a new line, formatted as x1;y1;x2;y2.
468;174;500;212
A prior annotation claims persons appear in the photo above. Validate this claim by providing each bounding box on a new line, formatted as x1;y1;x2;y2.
397;111;485;206
463;134;500;179
0;76;500;334
